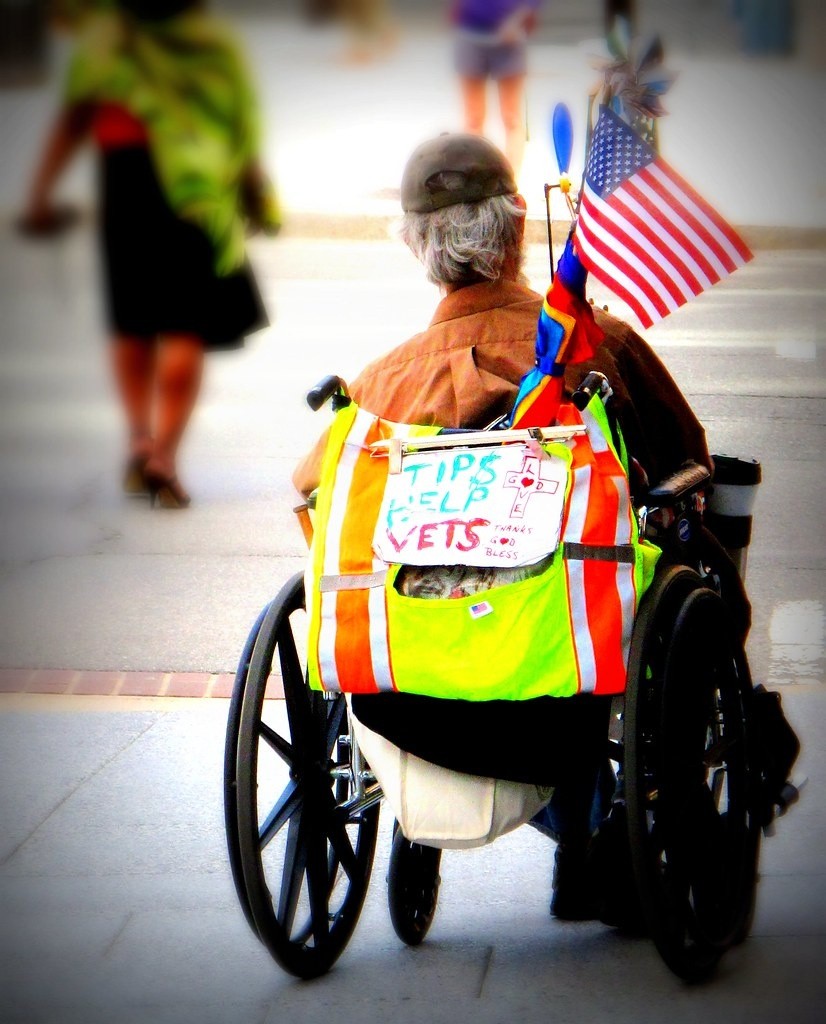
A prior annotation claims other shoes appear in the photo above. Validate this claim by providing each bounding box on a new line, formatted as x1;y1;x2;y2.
551;846;630;921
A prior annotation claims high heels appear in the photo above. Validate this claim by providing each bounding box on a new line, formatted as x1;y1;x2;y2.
122;453;191;509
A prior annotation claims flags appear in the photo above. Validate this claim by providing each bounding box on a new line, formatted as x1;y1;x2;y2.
572;105;755;331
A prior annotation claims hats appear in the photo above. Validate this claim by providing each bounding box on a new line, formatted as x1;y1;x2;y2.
401;132;518;211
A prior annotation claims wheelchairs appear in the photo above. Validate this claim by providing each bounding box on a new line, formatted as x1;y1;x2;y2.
221;373;809;992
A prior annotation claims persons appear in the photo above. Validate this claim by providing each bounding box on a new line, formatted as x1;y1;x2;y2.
443;0;541;176
23;0;283;509
291;133;714;935
